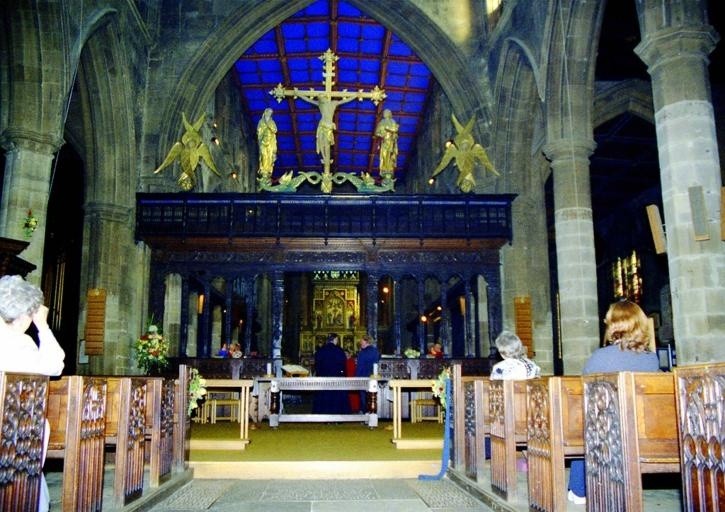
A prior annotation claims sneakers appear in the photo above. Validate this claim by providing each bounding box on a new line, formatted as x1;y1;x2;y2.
567;490;586;504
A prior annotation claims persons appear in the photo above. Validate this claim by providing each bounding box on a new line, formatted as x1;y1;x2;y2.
393;342;443;359
0;275;67;512
313;332;381;413
373;108;400;175
216;342;242;358
296;92;362;164
566;298;662;506
255;107;278;178
489;329;542;381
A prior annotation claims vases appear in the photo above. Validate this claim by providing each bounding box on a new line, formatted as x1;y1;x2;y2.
147;362;159;376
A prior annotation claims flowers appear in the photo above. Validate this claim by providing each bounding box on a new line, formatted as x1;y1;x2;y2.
133;315;169;366
188;369;204;413
432;368;450;407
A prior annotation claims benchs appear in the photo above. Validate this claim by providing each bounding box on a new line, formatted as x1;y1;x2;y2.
675;368;725;509
453;365;497;477
528;378;588;509
462;380;497;477
582;370;682;511
488;381;542;502
1;371;190;511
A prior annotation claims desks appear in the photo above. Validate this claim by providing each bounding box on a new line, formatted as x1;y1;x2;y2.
389;379;446;439
196;378;254;441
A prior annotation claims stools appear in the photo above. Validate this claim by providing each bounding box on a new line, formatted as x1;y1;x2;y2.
411;399;442;423
209;398;240;423
